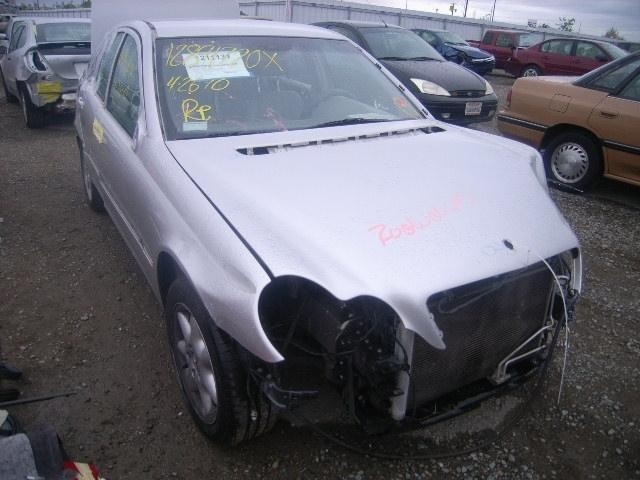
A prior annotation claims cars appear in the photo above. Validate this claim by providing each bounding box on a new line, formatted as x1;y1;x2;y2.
408;28;496;76
306;19;498;126
0;15;92;128
494;50;640;191
503;37;629;79
76;18;584;446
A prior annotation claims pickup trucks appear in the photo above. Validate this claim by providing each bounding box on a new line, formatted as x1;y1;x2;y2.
463;28;532;68
